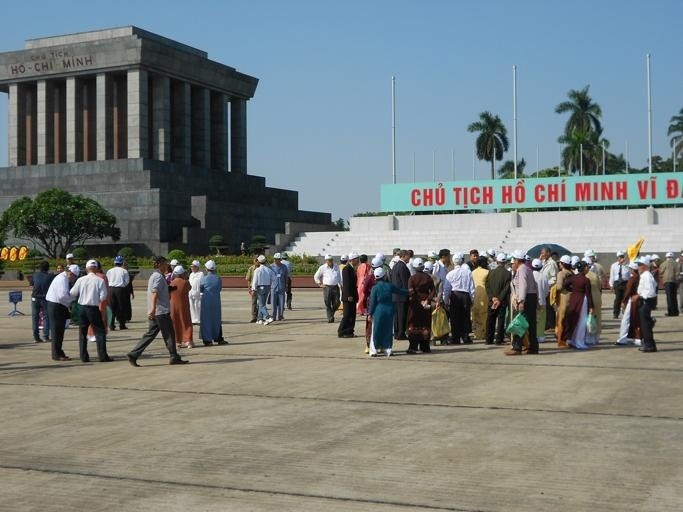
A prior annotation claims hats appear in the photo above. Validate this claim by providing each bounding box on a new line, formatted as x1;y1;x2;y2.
86;259;98;269
69;264;80;277
114;255;124;264
412;248;596;269
154;257;216;276
616;251;675;267
273;252;387;279
257;255;267;263
66;253;74;259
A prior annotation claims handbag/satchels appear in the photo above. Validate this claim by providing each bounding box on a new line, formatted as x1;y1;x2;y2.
587;312;599;335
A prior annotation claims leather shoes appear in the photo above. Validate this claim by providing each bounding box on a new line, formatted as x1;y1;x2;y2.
126;354;140;368
169;359;189;365
504;349;521;355
524;349;540;355
54;356;72;362
639;346;657;352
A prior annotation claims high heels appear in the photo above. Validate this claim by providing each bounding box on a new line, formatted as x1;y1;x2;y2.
387;349;393;358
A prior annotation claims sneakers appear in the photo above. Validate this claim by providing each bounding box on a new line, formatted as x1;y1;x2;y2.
256;320;263;325
264;318;274;326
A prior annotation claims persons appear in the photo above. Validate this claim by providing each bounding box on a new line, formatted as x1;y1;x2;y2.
166;258;229;348
251;255;273;326
246;258;266;323
30;253;135;362
280;253;292;311
127;258;189;367
338;247;606;358
610;249;683;352
269;253;289;321
313;255;342;322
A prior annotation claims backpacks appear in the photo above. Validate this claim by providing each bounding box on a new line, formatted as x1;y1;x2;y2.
430;306;451;339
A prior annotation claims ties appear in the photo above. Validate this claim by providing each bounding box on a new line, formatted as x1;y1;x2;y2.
618;264;623;284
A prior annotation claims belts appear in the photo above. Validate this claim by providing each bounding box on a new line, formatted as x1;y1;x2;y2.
110;286;121;289
